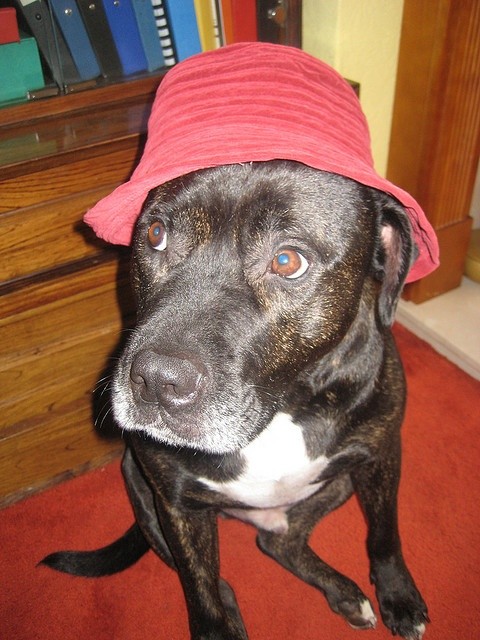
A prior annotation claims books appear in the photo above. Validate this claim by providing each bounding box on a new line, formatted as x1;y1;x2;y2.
17;0;279;89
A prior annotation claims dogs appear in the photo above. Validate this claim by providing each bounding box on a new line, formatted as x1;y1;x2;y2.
36;41;441;640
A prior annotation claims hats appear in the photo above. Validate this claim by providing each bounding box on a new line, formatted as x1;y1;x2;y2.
84;42;439;283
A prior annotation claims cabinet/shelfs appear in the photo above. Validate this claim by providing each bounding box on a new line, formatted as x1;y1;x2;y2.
0;0;365;503
